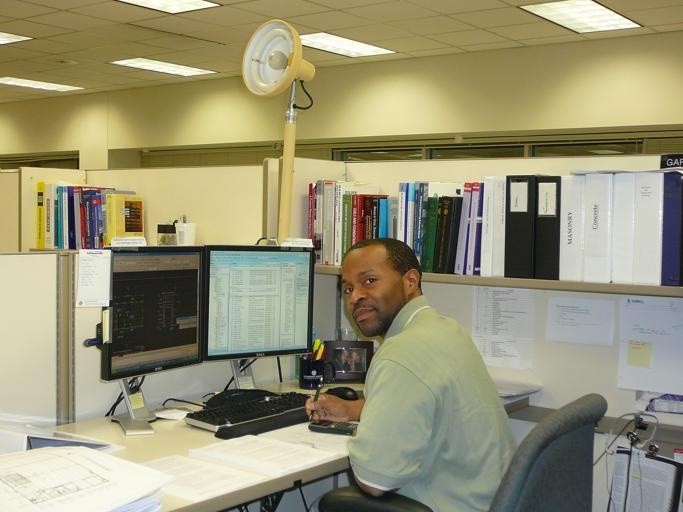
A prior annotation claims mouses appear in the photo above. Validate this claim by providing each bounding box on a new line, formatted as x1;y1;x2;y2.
328;383;360;403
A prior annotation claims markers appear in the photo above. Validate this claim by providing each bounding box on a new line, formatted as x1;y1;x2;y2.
308;339;325;362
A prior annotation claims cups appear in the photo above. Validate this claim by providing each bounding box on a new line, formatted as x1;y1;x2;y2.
299;358;335;389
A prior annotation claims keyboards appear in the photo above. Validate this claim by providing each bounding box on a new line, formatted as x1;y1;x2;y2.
187;391;312;439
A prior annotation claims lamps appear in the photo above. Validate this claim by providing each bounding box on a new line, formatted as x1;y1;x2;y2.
240;16;320;249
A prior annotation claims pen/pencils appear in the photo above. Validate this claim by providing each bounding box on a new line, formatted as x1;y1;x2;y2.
309;377;324;423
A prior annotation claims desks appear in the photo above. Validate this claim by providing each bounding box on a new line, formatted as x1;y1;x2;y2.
27;361;545;507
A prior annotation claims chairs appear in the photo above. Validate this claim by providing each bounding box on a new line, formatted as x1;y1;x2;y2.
308;393;610;507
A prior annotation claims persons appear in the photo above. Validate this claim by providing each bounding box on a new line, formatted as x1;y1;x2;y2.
331;349;363;372
304;238;517;512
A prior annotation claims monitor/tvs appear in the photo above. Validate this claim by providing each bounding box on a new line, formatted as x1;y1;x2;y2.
98;246;204;436
203;245;313;389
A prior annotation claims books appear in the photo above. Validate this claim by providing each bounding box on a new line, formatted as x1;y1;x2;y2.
305;177;484;275
35;178;145;249
